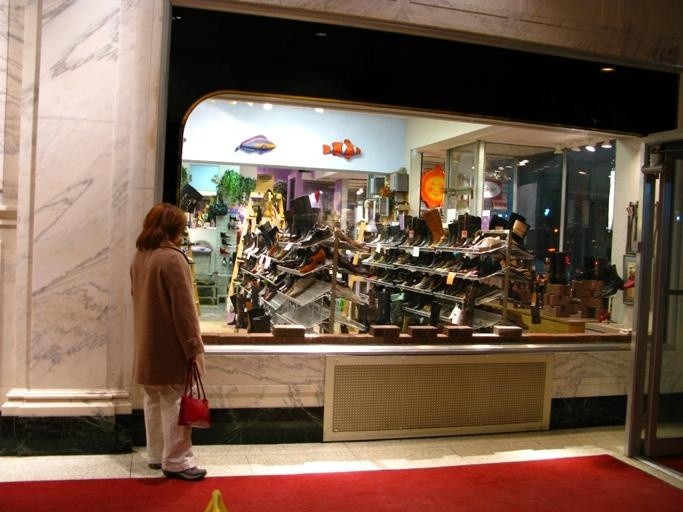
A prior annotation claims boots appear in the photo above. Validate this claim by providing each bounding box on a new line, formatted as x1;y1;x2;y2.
594;256;609;281
577;254;594;281
548;252;570;284
602;264;624;296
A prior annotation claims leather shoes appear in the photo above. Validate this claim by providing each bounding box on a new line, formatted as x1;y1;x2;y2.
213;196;332;333
619;271;636;289
335;226;363;248
367;209;537;332
336;254;364;275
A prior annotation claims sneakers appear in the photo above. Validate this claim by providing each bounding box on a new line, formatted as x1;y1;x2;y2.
147;457;161;470
160;459;208;481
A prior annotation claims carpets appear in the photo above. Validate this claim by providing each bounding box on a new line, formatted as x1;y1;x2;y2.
0;454;683;512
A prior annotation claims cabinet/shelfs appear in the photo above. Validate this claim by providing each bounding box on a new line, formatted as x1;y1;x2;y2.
180;141;567;334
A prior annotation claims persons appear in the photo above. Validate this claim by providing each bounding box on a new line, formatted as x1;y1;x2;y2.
127;200;209;482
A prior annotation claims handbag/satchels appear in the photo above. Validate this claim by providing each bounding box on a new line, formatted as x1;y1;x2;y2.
176;358;212;431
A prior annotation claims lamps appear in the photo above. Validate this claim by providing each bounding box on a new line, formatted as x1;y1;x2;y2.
554;136;613;154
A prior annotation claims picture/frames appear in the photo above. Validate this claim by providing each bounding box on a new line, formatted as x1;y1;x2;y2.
623;255;636;305
